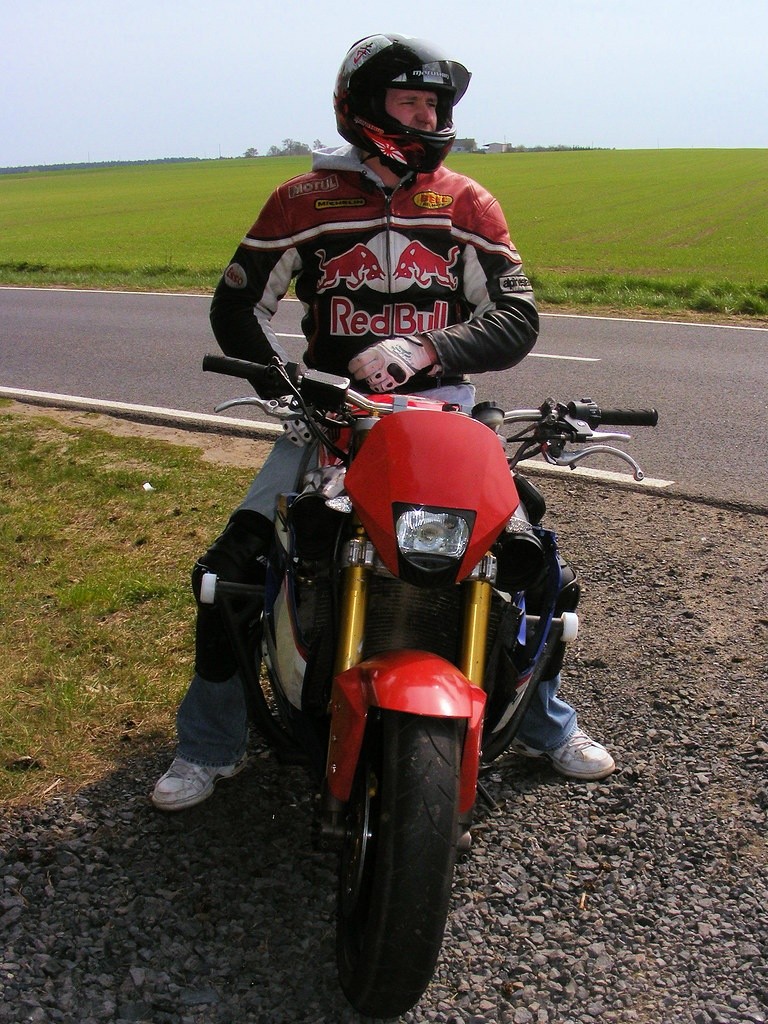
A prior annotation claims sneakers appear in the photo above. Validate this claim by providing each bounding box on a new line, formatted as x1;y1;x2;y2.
152;751;248;810
511;724;616;779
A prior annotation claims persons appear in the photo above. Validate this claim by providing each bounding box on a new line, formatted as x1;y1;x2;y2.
149;29;620;811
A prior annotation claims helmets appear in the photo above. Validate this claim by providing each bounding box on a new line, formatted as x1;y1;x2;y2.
332;32;472;173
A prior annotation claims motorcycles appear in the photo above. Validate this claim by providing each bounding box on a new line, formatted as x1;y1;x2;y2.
201;354;658;1018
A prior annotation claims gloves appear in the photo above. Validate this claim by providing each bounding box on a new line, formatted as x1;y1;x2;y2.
273;394;323;447
349;335;432;393
